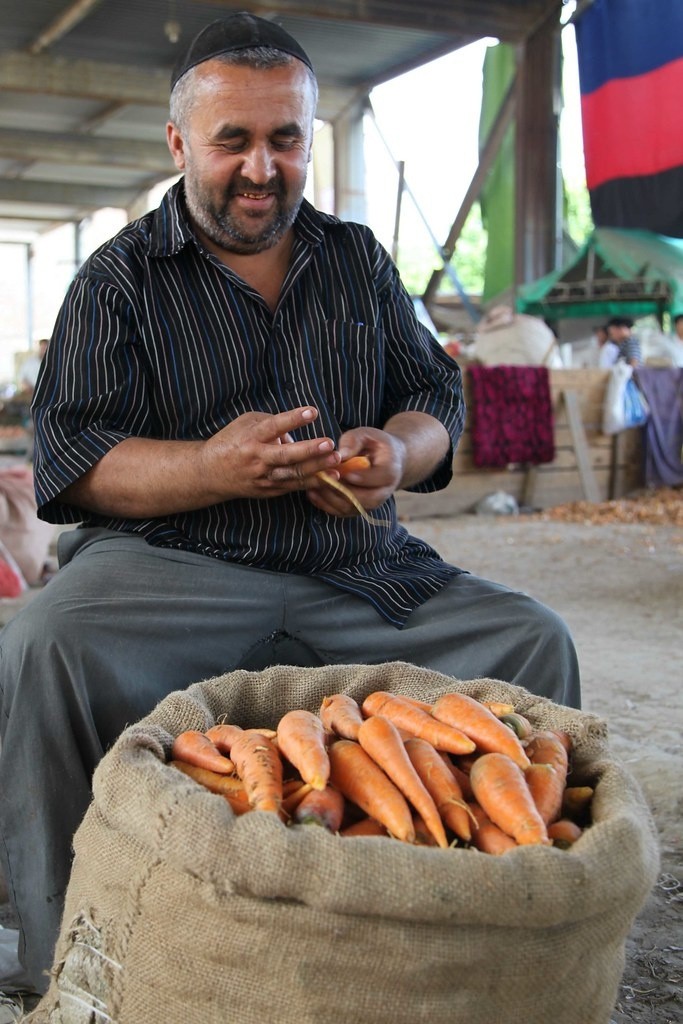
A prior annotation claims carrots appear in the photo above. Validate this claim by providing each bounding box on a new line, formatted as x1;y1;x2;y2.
167;690;592;857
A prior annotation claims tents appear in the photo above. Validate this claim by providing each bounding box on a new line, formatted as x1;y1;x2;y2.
512;228;683;345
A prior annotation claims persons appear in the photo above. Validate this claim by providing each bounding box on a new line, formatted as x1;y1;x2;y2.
594;313;683;368
0;12;582;1024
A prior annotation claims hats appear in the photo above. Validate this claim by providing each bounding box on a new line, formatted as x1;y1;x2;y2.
171;13;314;92
